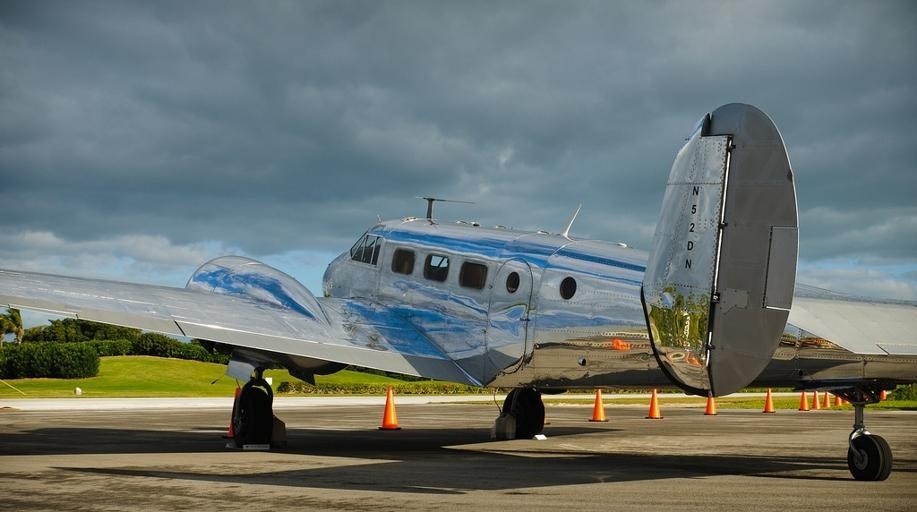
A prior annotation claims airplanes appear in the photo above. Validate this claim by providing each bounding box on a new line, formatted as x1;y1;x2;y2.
0;105;913;478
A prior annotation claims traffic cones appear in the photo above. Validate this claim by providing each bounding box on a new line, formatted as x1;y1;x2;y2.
879;390;887;401
226;387;240;438
380;383;400;428
647;389;662;418
799;390;848;411
707;392;715;414
589;386;608;421
763;386;775;414
